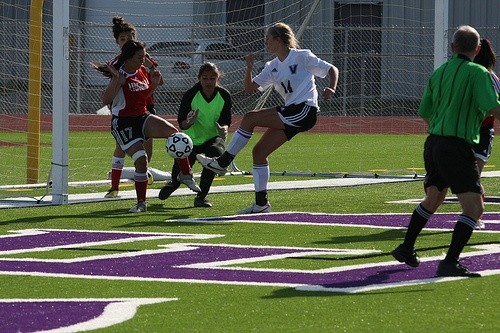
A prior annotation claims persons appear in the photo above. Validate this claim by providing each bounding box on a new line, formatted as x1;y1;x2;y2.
90;40;201;213
469;40;500;230
391;26;500;278
104;16;164;198
196;23;338;214
159;62;233;207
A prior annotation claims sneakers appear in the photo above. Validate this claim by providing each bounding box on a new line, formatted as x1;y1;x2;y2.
196;154;228;176
437;259;481;277
128;202;147;213
146;170;154;185
392;244;422;268
104;190;118;198
177;171;202;192
238;201;272;214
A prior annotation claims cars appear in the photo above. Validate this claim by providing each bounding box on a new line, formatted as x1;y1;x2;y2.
145;40;266;95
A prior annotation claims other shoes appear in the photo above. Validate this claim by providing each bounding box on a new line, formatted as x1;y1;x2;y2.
158;181;180;200
474;219;485;230
194;197;213;208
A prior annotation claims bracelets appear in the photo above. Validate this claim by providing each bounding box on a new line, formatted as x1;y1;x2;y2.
149;63;154;69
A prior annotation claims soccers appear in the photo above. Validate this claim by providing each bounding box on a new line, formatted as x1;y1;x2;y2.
165;132;194;159
